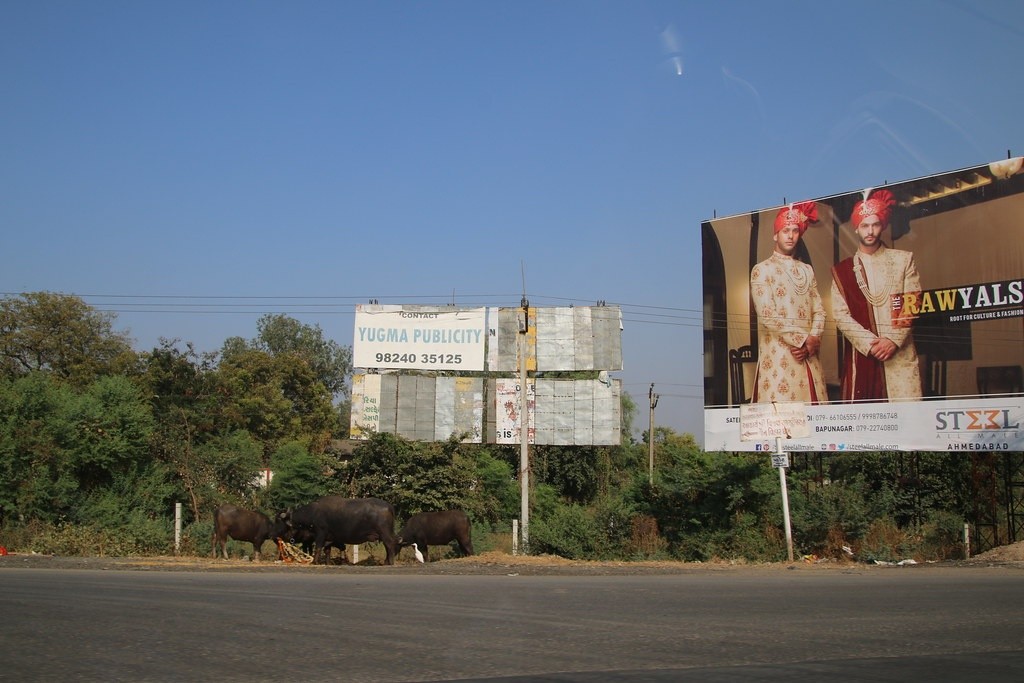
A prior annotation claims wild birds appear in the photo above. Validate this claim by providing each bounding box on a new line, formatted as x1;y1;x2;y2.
410;542;425;565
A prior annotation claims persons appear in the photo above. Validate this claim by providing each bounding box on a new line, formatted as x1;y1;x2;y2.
750;203;829;405
831;190;923;403
335;547;348;563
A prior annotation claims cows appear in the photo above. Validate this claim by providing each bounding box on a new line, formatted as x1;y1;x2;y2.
395;509;473;563
212;497;394;566
